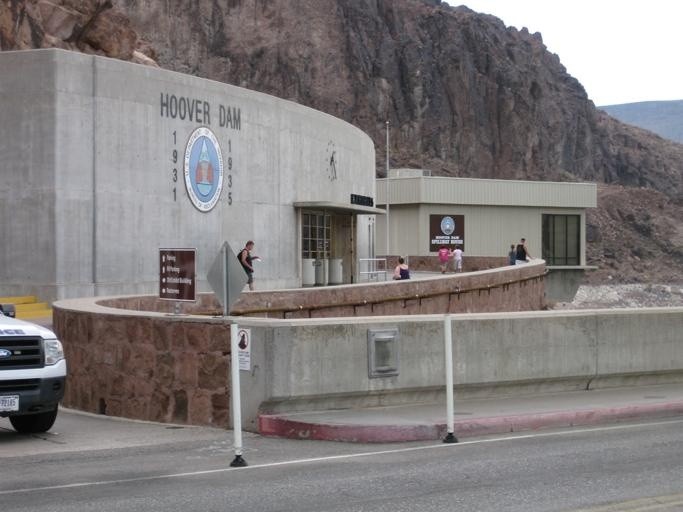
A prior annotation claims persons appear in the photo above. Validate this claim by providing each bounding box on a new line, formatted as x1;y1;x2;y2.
393;254;410;281
438;243;451;274
451;244;463;275
514;237;534;264
236;240;259;289
507;243;516;263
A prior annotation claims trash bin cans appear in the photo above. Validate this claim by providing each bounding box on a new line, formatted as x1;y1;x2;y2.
302;258;343;287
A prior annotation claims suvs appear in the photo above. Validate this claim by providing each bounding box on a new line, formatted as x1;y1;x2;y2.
1;303;68;434
